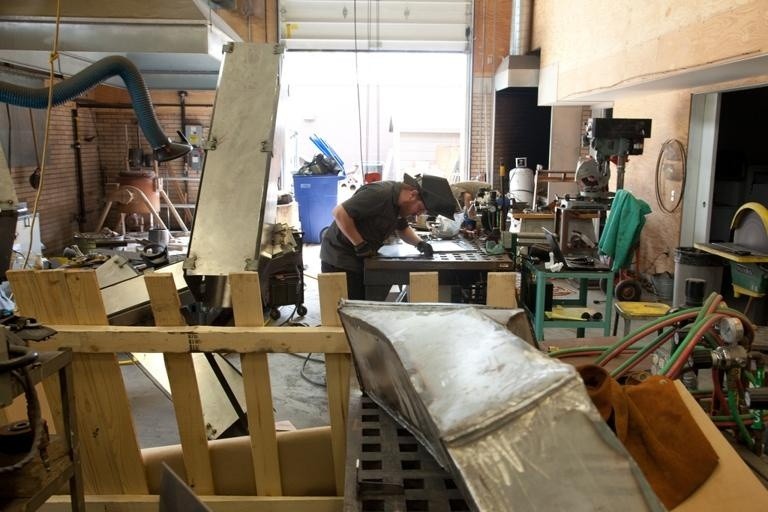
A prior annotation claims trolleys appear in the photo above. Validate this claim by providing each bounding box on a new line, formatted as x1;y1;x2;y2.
259;222;307;320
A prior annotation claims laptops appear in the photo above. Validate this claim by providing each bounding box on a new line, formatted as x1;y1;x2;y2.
541;227;610;271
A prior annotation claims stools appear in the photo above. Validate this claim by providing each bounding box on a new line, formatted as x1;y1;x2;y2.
613;302;671;337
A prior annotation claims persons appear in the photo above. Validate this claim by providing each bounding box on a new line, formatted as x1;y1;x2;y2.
320;178;438;303
450;181;491;211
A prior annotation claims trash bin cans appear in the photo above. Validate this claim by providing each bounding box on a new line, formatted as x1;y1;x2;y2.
292;133;346;243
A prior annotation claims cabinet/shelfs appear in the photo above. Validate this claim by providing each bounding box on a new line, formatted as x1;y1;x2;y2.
0;346;85;512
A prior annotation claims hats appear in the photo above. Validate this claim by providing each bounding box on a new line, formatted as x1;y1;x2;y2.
404;172;462;221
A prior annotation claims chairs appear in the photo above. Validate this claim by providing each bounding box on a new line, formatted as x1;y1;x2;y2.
520;189;651;342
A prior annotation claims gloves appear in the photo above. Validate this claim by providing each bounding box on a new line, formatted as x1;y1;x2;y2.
417;241;433;256
354;240;376;257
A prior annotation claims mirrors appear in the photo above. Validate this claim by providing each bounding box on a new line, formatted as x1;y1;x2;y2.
654;138;686;214
